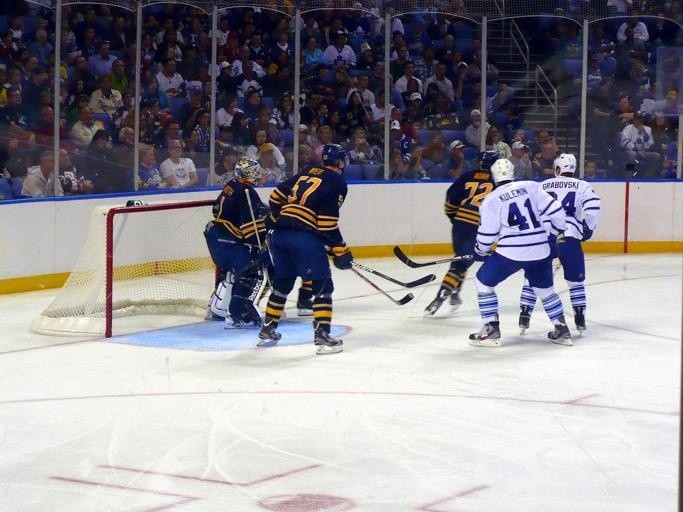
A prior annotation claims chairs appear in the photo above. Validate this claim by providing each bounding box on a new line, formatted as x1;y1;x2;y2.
0;0;683;200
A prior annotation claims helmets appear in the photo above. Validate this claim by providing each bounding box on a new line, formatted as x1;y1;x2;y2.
234;156;262;187
321;143;349;168
490;158;514;183
477;150;498;172
552;152;576;178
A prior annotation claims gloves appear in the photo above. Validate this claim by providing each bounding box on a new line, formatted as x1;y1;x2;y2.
581;219;592;242
472;243;488;262
331;243;353;269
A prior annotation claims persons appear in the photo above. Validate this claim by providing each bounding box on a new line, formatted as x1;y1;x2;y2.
258;259;350;308
469;159;570;340
0;0;683;200
425;151;499;314
519;153;600;327
205;158;265;323
258;143;354;345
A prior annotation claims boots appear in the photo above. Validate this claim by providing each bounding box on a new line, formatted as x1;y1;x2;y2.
424;288;450;315
297;287;313;309
258;316;281;340
548;314;571;340
312;319;343;346
519;305;531;329
574;306;585;327
469;314;501;341
451;291;461;304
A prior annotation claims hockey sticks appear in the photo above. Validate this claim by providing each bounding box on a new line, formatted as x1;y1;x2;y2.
394;246;474;269
324;245;436;288
351;268;414;305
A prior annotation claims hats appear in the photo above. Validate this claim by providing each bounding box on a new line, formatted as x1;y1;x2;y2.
449;139;467;150
456;60;468;71
512;141;529;150
260;143;274;154
298;123;308;132
390;120;401;131
410;92;422;101
